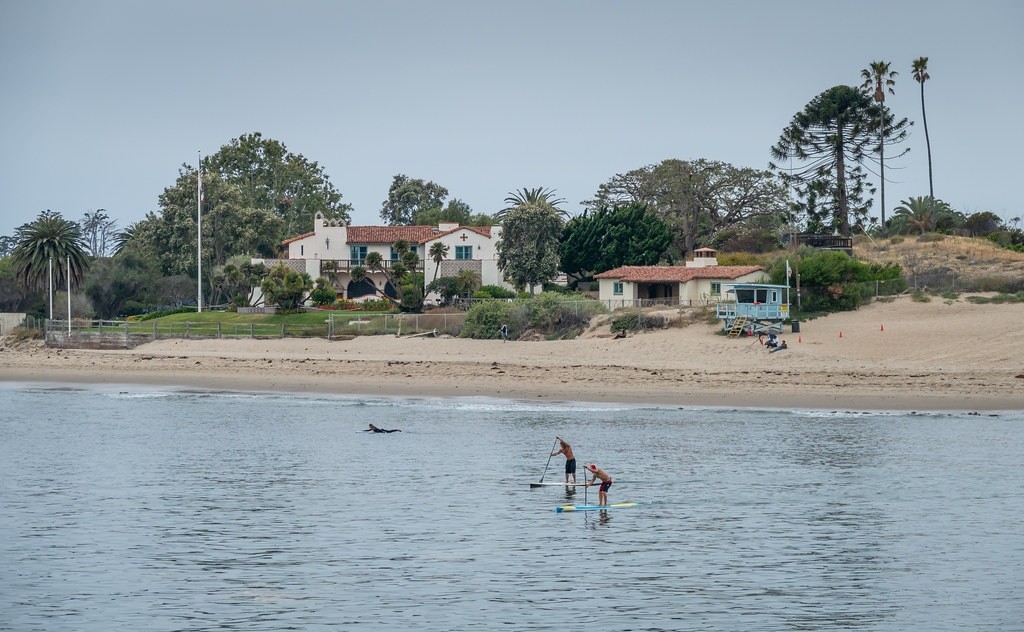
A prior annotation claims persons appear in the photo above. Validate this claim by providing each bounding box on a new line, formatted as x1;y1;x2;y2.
550;437;576;483
764;331;787;354
583;465;612;506
614;329;627;339
499;321;507;343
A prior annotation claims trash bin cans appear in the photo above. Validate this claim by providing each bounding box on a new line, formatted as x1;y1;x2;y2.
790;319;799;332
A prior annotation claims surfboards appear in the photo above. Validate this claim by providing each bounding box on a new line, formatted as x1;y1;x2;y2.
530;482;604;486
556;502;644;512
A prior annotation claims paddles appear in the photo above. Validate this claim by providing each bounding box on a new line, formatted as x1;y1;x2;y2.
583;468;588;506
538;439;557;483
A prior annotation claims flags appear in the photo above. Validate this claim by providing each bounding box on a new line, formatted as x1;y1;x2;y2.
786;261;792;279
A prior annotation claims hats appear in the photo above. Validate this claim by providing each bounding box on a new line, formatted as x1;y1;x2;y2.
589;464;596;470
